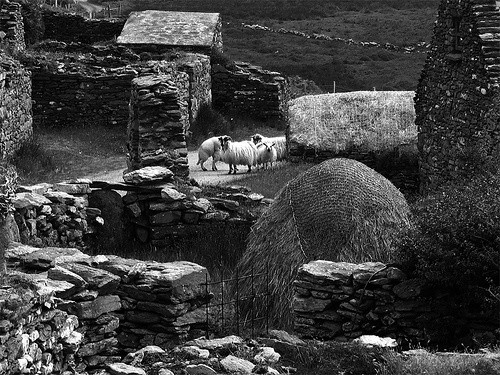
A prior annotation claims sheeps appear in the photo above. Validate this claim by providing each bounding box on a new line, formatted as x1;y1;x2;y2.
195;134;285;174
